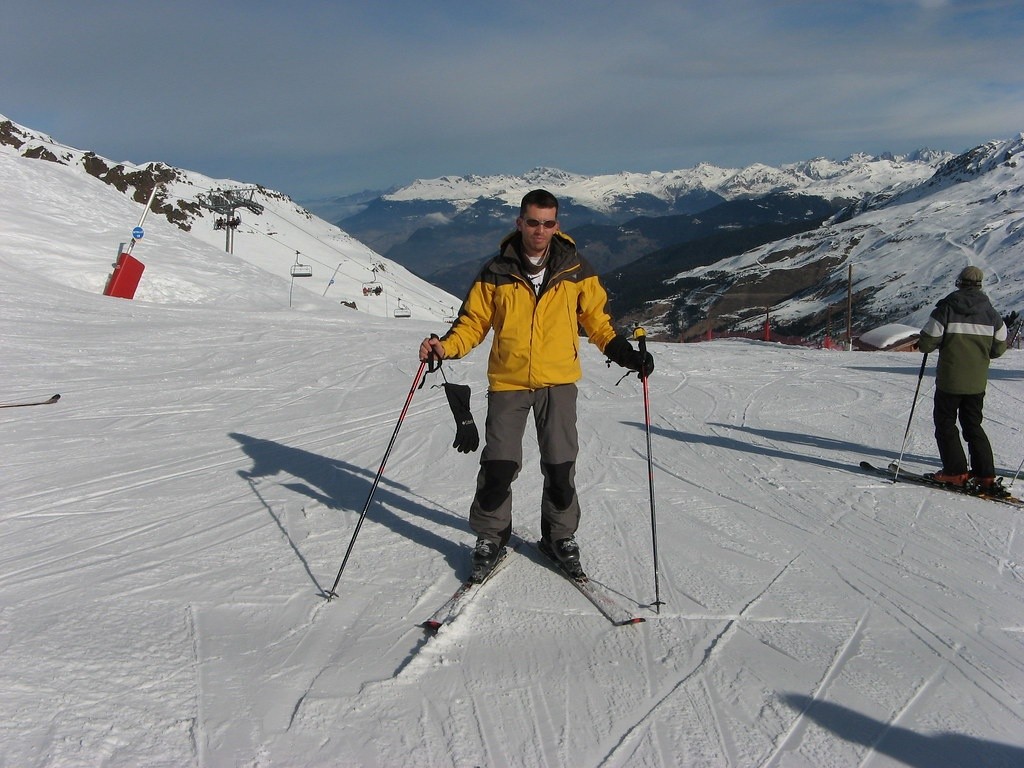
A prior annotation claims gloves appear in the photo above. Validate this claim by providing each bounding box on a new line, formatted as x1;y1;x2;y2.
445;383;479;454
603;334;654;382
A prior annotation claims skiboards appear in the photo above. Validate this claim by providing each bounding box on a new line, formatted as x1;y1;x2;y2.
856;459;1024;510
421;532;648;635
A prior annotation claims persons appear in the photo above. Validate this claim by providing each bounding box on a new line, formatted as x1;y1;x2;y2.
214;217;242;230
421;189;655;586
363;286;382;296
918;265;1008;489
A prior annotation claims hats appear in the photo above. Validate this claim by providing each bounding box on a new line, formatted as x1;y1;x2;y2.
959;266;983;281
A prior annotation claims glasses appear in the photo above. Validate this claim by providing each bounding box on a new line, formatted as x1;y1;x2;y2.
521;217;556;228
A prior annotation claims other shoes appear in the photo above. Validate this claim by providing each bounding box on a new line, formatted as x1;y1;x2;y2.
934;470;968;487
968;471;993;489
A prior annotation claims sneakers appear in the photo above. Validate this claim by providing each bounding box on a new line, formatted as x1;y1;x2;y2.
541;536;580;563
470;536;503;570
